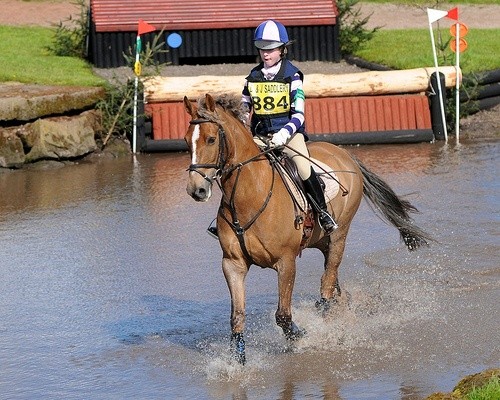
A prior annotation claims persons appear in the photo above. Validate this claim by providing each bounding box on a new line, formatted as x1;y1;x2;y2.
206;21;340;239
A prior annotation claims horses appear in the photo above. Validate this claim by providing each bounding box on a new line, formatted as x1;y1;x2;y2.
182;93;441;365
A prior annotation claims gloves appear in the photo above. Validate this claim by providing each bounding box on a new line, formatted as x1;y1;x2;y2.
271;128;292;149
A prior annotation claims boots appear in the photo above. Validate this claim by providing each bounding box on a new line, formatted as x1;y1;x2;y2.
297;165;339;234
206;227;219;239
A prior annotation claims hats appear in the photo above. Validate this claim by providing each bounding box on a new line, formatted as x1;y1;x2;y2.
252;19;289;50
